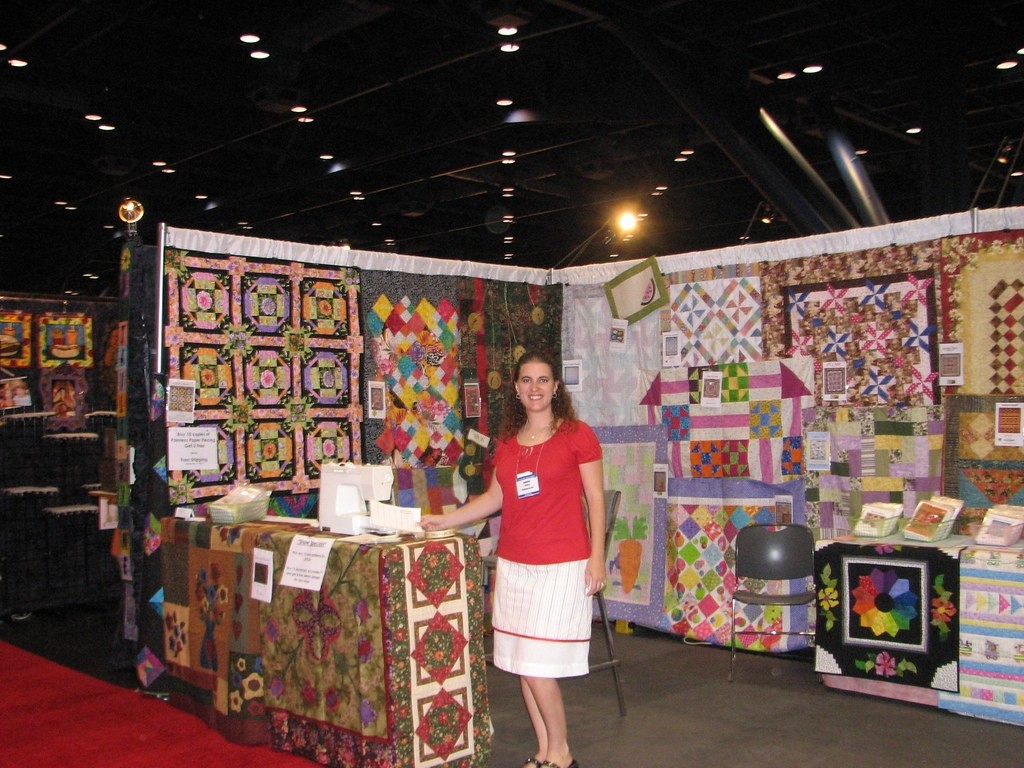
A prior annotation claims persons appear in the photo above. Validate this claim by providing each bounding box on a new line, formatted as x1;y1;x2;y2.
416;349;606;768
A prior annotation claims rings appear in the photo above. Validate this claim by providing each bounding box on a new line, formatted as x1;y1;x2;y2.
601;583;604;586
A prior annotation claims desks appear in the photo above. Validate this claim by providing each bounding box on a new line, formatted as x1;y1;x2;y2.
815;531;1024;728
161;517;493;768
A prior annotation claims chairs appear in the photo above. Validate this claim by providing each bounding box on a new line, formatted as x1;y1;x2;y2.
587;490;629;718
727;522;819;686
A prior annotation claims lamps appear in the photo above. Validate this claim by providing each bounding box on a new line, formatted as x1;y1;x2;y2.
117;197;145;238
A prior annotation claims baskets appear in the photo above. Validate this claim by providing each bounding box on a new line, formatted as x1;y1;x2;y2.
848;513;902;538
206;497;270;525
900;516;960;542
966;521;1024;547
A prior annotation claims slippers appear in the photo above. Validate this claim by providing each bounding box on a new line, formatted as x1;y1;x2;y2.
526;758;541;768
543;758;579;768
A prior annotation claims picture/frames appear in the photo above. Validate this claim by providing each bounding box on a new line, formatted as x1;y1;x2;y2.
662;332;681;366
938;343;964;386
823;361;847;401
117;321;128;418
806;431;830;471
35;314;95;368
368;380;386;419
561;360;582;392
464;383;482;418
0;376;36;411
603;254;671;326
995;402;1024;446
701;372;722;407
0;311;33;368
40;366;89;431
166;378;196;424
852;496;1024;547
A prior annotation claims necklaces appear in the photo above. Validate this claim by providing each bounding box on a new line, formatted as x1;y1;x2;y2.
531;436;535;439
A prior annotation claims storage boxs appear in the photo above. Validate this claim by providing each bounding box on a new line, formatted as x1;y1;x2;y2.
204;498;270;525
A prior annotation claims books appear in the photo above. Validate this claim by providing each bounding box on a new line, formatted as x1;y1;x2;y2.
976;504;1024;546
853;503;903;536
904;496;963;541
